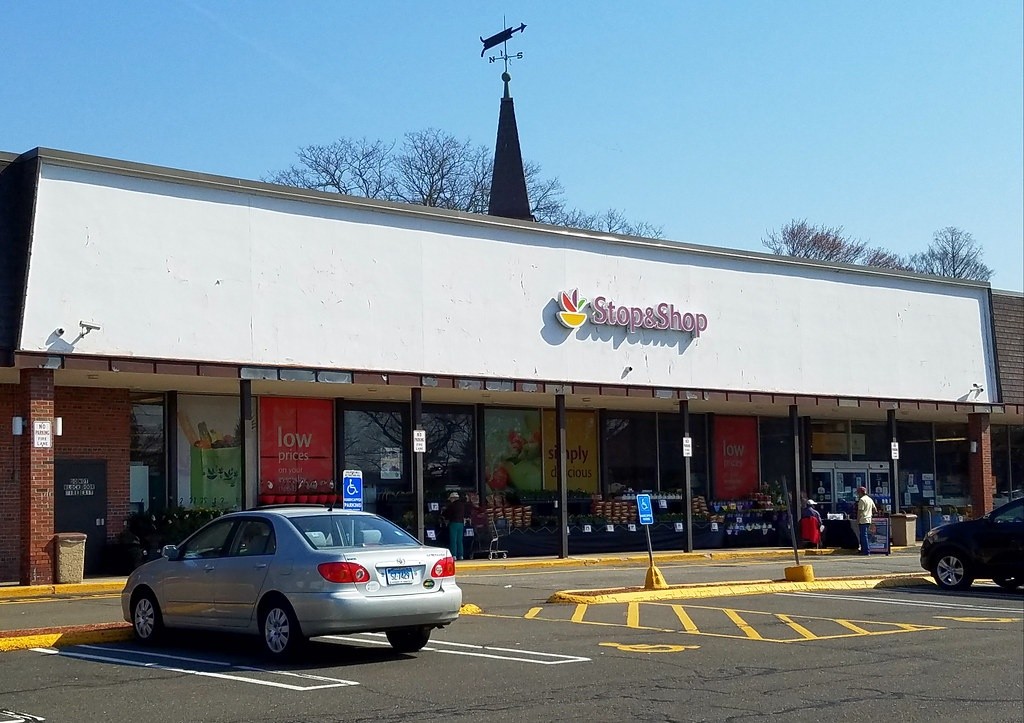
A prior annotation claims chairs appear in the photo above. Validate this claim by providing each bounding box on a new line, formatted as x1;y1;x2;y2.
799;516;823;549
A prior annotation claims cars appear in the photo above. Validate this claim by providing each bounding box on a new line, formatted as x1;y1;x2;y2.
920;496;1024;593
120;503;462;665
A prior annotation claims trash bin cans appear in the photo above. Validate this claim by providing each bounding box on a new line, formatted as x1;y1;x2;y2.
890;513;918;547
55;532;87;585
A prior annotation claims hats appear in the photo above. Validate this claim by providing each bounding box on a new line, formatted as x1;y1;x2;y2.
855;486;867;493
806;499;818;505
447;492;459;500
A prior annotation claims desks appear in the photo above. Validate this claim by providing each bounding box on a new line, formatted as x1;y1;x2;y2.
820;519;861;549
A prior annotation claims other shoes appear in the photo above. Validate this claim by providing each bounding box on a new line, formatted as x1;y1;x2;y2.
858;551;871;556
819;545;826;549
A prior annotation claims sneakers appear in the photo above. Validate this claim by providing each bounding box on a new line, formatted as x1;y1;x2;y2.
458;556;463;560
453;556;457;561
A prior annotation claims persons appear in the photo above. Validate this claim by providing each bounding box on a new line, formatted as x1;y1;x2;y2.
241;524;263;550
801;499;822;548
443;493;465;560
856;487;877;557
